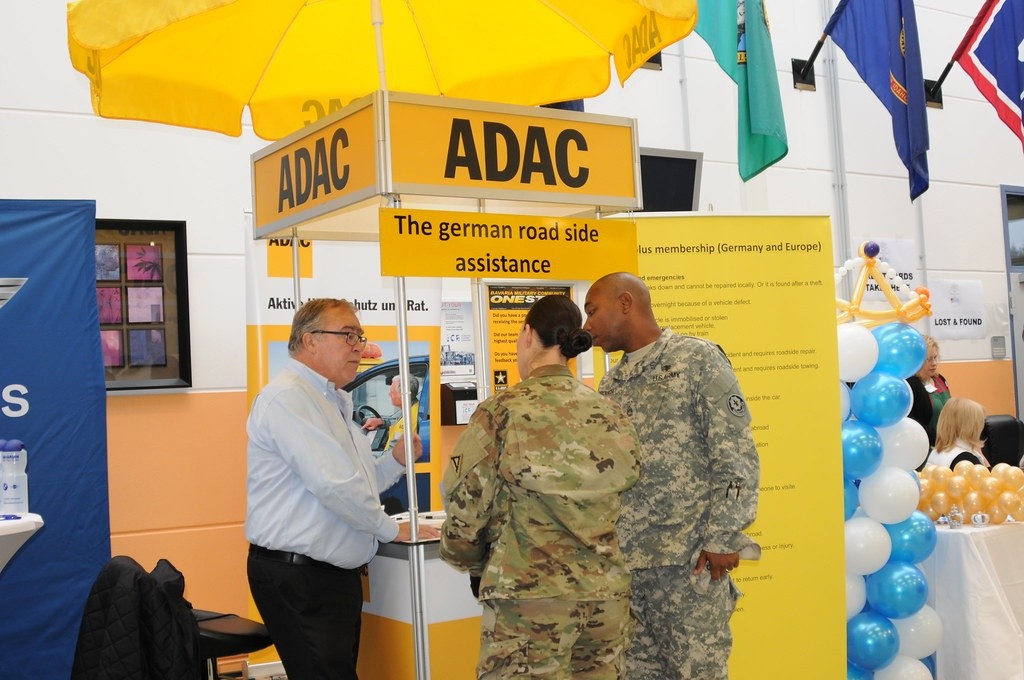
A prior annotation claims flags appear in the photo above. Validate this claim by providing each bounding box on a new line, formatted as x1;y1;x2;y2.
693;0;789;182
826;0;930;201
953;0;1024;151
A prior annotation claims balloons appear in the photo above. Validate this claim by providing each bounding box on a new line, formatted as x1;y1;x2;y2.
836;322;879;382
834;239;934;328
870;323;926;378
837;372;942;680
913;461;1024;525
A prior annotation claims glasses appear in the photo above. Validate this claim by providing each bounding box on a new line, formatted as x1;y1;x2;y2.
309;328;368;350
927;356;940;363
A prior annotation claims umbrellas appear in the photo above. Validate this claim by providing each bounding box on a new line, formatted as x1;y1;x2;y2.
65;0;697;139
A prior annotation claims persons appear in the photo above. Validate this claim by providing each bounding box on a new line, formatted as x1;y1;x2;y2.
439;295;643;680
927;397;992;472
583;272;761;680
245;298;444;680
907;334;952;450
361;374;420;451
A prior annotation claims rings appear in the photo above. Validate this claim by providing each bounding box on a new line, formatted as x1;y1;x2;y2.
726;569;731;574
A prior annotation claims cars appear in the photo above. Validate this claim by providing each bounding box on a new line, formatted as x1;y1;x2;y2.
340;355;432;518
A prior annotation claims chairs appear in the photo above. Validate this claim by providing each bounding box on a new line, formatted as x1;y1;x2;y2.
69;555;274;680
978;415;1024;467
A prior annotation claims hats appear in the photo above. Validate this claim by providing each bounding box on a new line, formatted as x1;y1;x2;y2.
689;532;763;595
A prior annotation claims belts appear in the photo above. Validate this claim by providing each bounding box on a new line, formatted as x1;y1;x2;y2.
249;545;369;576
